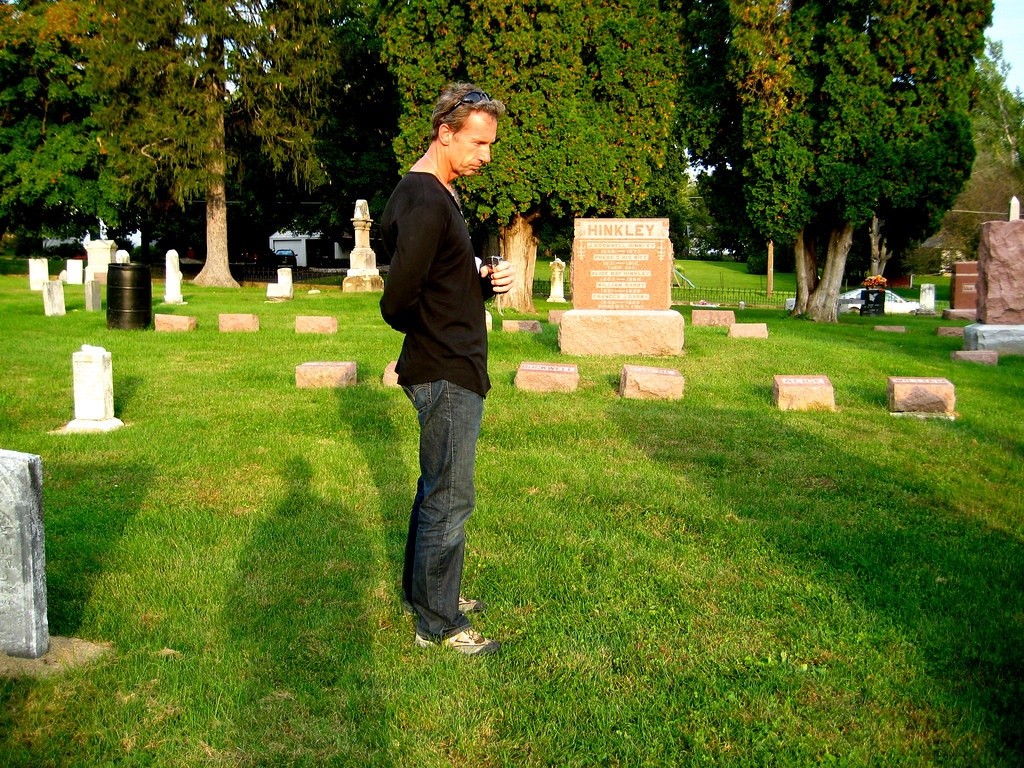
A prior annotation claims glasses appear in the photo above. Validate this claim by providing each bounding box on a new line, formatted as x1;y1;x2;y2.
441;92;491;117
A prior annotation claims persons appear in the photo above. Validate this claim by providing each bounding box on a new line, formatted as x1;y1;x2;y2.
378;83;516;655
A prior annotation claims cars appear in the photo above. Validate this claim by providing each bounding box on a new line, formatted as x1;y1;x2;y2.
784;288;920;314
274;249;299;270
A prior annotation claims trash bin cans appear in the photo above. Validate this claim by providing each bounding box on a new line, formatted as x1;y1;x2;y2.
106;262;152;330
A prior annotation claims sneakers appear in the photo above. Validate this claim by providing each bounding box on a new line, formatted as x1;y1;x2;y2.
415;621;501;656
458;597;483;612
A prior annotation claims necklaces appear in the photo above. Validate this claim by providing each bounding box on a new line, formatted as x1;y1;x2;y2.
425;153;447;187
